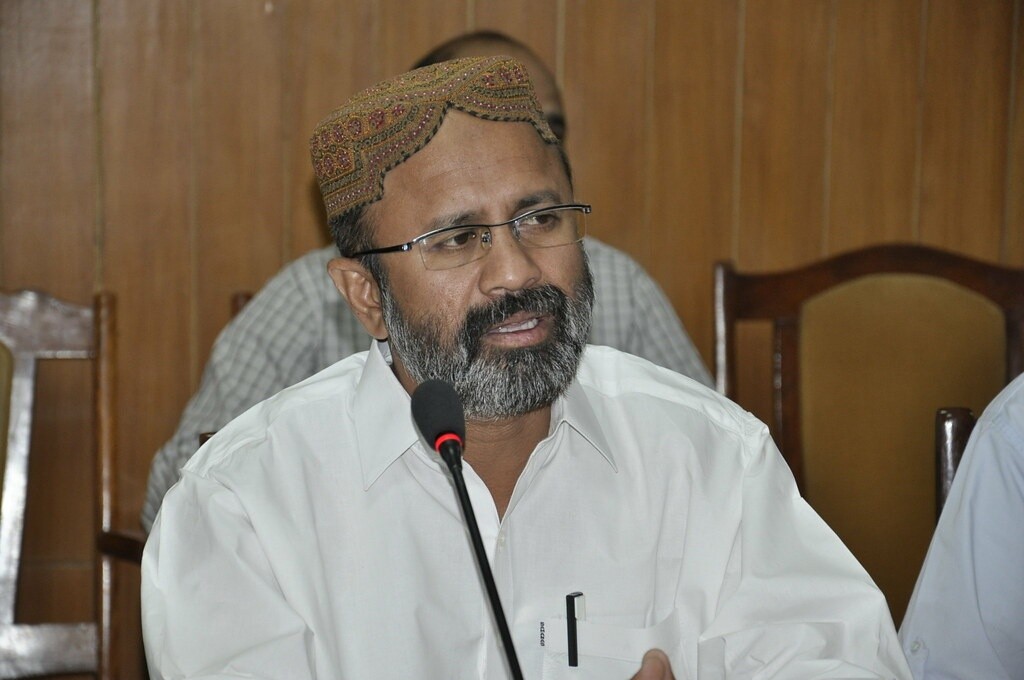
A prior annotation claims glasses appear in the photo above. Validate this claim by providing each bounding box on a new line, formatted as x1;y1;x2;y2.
344;204;592;271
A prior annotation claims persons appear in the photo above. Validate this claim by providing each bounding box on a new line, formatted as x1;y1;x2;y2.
898;373;1024;679
140;54;912;679
142;30;716;535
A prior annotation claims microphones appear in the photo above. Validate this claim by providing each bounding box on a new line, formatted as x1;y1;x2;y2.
411;379;526;680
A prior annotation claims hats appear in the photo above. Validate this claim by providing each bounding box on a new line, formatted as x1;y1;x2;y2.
309;57;559;240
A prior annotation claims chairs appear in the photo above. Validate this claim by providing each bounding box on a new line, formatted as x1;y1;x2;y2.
712;243;1024;532
0;284;254;680
